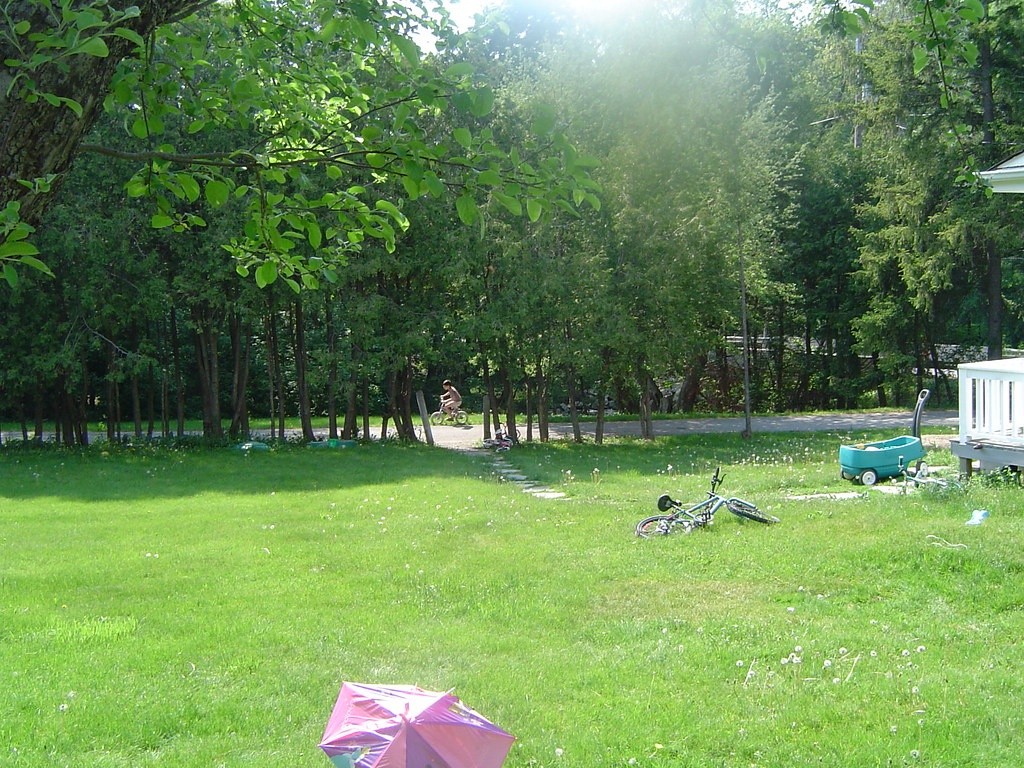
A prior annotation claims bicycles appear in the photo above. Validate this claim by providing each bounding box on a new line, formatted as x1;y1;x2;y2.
483;422;520;453
429;395;468;425
633;466;780;542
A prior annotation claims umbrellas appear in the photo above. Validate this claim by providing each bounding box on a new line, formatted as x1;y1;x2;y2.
318;681;517;768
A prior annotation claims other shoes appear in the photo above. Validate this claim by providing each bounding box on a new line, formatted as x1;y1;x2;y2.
446;418;454;421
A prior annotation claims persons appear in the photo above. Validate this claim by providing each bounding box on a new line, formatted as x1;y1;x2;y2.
440;380;462;421
